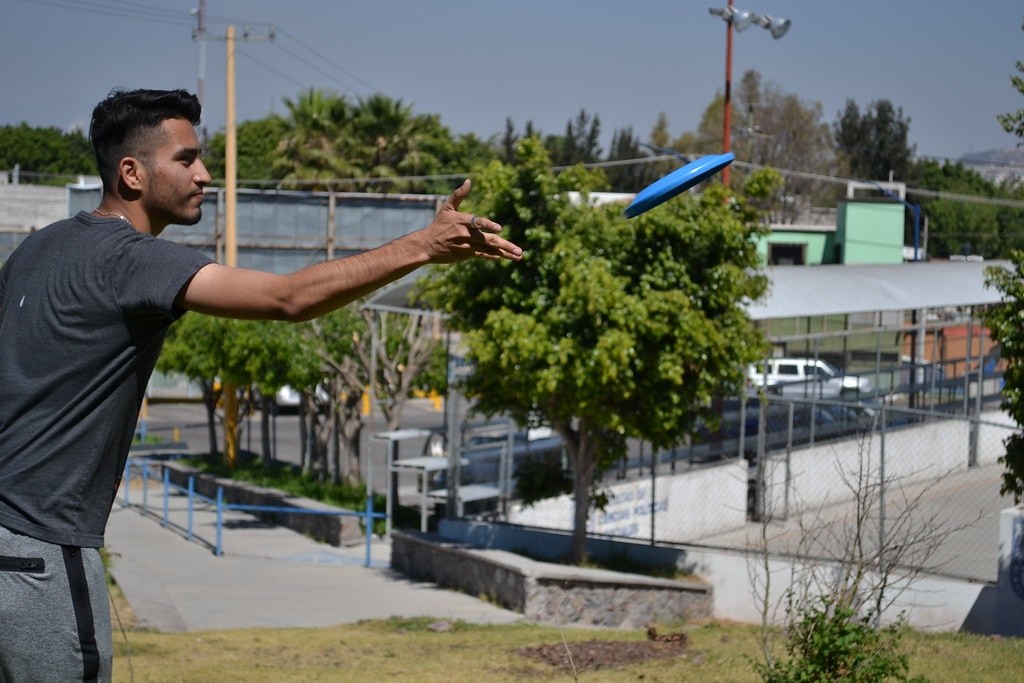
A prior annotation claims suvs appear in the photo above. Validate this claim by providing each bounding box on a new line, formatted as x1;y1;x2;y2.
747;359;870;402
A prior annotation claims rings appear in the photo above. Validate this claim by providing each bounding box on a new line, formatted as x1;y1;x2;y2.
471;215;478;230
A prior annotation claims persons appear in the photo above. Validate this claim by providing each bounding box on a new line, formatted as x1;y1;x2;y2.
0;88;523;683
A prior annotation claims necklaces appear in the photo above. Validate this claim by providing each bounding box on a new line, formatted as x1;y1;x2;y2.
94;208;132;227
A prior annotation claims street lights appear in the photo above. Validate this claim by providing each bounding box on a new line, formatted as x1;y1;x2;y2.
707;1;790;265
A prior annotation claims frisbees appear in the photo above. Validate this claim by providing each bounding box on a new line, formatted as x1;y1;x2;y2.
622;150;738;220
